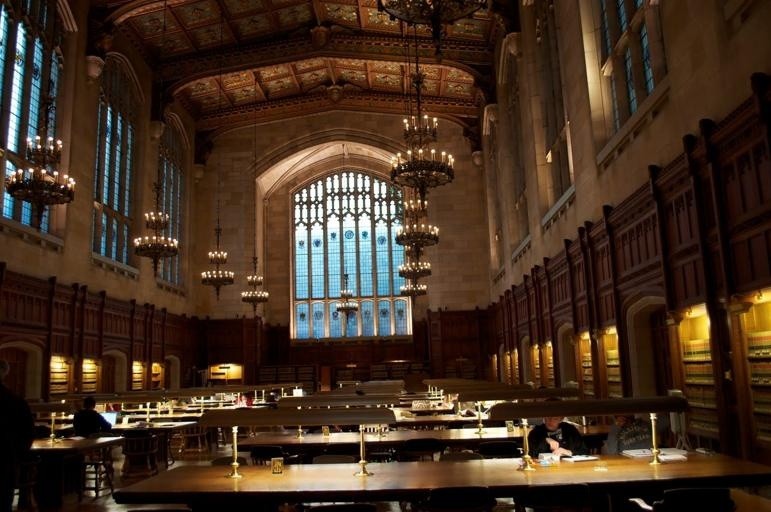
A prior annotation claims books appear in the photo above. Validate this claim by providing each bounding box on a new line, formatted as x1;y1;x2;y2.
621;448;652;458
651;446;689;454
562;454;599;462
658;454;688;463
538;450;574;463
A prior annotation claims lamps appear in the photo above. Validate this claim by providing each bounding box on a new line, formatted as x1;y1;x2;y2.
201;229;239;302
24;389;199;408
132;179;184;277
422;377;692;427
8;95;77;226
337;273;359;323
199;380;406;425
389;5;458;307
241;252;271;316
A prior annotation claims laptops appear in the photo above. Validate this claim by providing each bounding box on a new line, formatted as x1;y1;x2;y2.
99;412;117;426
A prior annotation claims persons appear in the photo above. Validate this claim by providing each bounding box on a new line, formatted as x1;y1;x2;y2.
75;398;112;436
603;411;678;454
237;393;253;406
525;398;590;455
0;358;35;511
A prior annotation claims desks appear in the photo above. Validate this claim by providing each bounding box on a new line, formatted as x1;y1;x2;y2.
0;373;771;512
